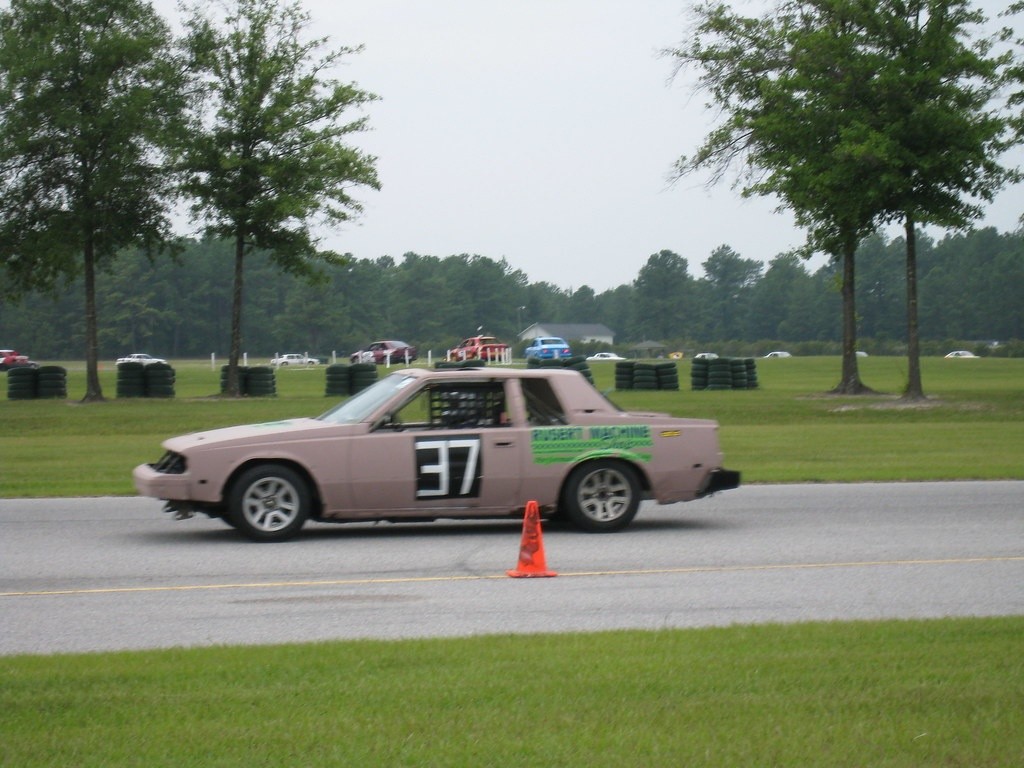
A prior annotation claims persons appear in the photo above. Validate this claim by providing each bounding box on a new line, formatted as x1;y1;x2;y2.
440;391;480;429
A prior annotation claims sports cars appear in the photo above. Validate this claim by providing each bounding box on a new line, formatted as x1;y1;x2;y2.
130;358;744;544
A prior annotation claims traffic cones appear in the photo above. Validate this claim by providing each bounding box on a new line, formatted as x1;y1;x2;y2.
503;501;558;579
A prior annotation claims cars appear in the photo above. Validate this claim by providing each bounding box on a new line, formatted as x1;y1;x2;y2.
944;351;981;358
272;354;320;367
350;340;418;365
525;336;573;363
115;353;168;367
444;337;508;361
585;352;626;360
763;351;792;358
694;352;719;359
0;350;38;371
655;351;683;359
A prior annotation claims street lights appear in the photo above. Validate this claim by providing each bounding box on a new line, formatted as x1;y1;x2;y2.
518;306;526;333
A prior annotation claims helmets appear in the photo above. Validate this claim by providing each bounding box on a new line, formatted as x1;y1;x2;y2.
440;383;486;424
